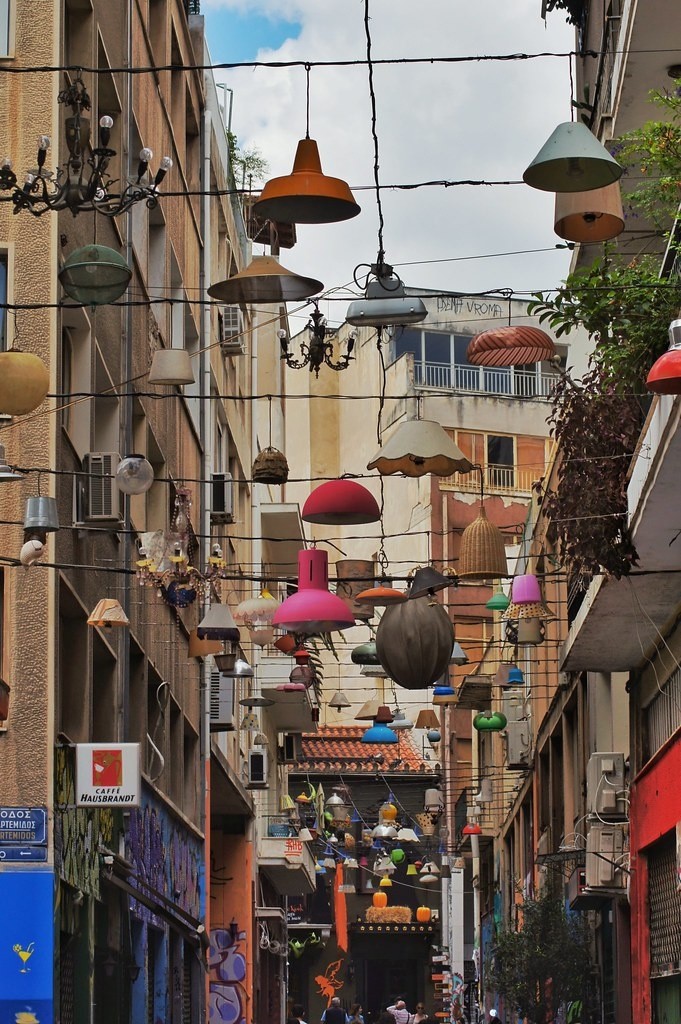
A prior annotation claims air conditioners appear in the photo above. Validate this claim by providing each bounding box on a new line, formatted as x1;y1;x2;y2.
211;669;236;730
222;303;246;355
585;825;623;888
248;748;269;785
503;721;529;766
283;734;298;764
501;690;524;721
81;451;127;530
586;751;625;815
210;472;238;525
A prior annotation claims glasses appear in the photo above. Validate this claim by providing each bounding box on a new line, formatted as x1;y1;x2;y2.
417;1006;424;1010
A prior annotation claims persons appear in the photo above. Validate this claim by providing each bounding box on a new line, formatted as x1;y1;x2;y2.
320;997;349;1024
488;1009;502;1024
286;1004;307;1024
348;1004;364;1024
386;996;440;1024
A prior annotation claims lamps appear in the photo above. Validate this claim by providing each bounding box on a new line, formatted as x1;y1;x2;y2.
367;400;474;478
0;310;50;416
136;534;558;926
276;302;360;379
465;297;557;367
523;56;625;192
22;474;60;534
147;303;196;386
87;570;130;634
207;245;324;301
344;278;429;327
57;208;133;306
554;180;626;243
458;468;508;581
301;481;381;526
645;318;681;395
252;65;362;225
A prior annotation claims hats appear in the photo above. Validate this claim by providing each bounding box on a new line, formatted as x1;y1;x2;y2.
291;1004;305;1013
